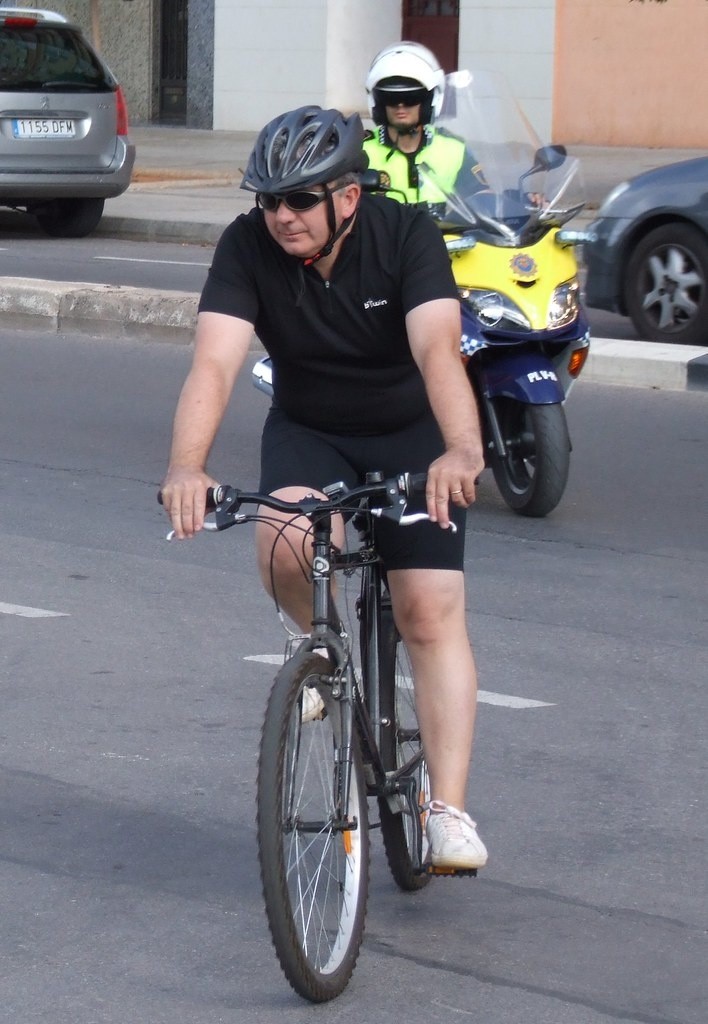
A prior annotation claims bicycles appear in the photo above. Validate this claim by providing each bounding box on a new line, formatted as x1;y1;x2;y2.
157;467;481;1004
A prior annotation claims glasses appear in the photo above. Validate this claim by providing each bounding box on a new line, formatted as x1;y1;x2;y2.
258;180;356;211
378;93;423;106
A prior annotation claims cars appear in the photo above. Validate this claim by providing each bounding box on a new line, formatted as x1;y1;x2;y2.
581;155;708;348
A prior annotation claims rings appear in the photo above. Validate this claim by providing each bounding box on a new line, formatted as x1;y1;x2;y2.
450;489;461;496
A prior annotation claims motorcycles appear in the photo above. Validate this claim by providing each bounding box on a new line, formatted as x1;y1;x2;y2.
249;67;599;518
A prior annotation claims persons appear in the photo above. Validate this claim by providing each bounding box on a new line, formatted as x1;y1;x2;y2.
160;105;495;870
362;42;550;233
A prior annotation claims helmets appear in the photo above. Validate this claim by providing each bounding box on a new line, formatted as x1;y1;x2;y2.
364;40;445;126
239;105;370;193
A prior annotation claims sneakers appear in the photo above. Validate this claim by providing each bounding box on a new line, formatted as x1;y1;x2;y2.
426;800;488;868
296;647;330;725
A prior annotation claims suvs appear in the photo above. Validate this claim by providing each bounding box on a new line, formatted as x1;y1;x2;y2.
0;6;138;238
